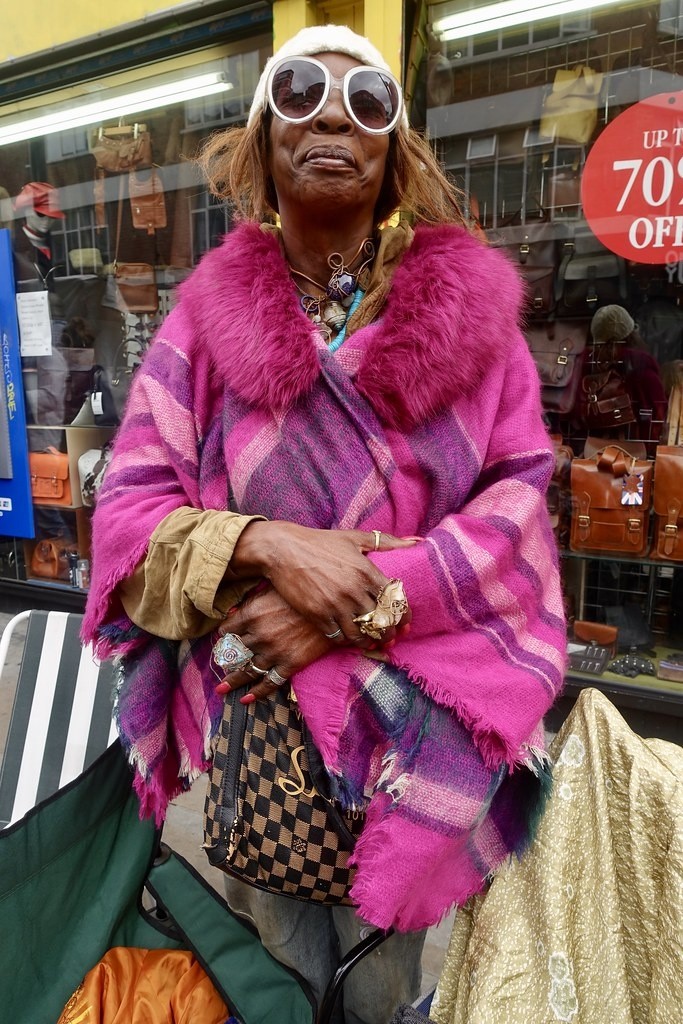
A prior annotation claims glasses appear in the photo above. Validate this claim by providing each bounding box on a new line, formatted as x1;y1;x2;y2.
117;312;161;370
266;54;403;134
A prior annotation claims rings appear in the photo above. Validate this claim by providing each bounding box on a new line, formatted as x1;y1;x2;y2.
372;579;408;630
249;660;268;675
372;530;382;551
353;610;386;639
211;634;256;672
269;667;287;686
325;627;343;639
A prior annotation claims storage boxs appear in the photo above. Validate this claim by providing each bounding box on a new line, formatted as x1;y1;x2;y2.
22;504;94;590
23;428;115;509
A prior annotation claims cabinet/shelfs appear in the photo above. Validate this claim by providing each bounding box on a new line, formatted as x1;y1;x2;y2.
545;549;683;748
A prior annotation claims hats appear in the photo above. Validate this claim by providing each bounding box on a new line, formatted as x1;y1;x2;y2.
591;304;634;344
14;182;65;221
246;23;410;132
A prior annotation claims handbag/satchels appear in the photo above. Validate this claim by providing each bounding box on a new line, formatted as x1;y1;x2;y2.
93;133;150;172
112;262;159;314
128;168;166;230
198;655;365;913
77;441;117;507
29;446;73;506
30;535;78;581
479;23;683;558
39;331;94;370
91;338;146;425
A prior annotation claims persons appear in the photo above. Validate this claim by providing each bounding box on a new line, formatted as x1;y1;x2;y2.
77;20;571;1024
568;305;669;454
10;182;79;448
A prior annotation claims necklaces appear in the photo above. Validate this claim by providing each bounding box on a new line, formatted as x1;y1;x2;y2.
287;234;379;352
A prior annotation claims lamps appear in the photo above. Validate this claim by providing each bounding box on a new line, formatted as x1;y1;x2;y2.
0;56;237;148
426;0;622;44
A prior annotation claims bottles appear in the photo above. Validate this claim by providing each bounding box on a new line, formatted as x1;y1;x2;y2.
69;551;90;590
